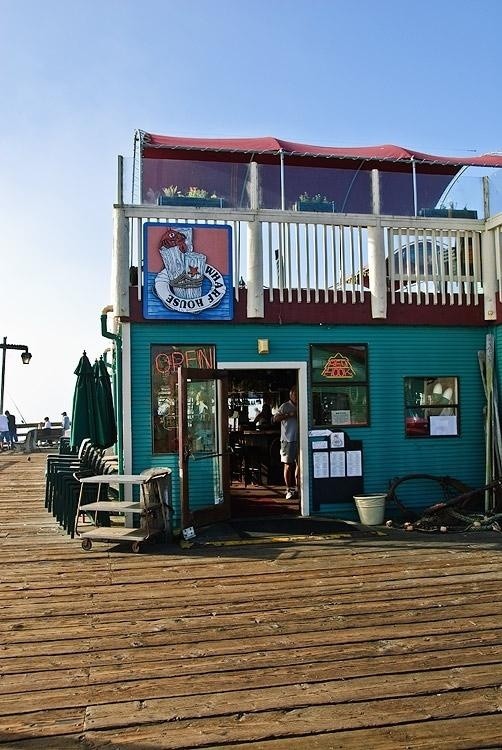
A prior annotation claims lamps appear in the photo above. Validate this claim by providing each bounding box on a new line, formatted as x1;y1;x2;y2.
433;378;453;400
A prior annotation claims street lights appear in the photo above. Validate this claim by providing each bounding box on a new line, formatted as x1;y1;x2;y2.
0;336;31;416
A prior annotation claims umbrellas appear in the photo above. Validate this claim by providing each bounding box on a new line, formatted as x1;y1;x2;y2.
69;349;117;450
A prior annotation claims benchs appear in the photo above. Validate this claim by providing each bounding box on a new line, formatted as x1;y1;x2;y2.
12;427;65;454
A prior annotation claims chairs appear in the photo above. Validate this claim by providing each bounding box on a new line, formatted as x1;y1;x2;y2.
44;436;114;540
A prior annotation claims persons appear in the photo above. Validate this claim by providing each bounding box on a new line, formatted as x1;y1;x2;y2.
4;410;19;443
43;416;52;445
269;384;300;498
0;415;11;450
61;411;71;431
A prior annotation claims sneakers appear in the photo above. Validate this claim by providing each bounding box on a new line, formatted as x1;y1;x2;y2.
285;488;298;501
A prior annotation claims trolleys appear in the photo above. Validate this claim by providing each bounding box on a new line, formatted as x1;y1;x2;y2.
69;468;170;553
